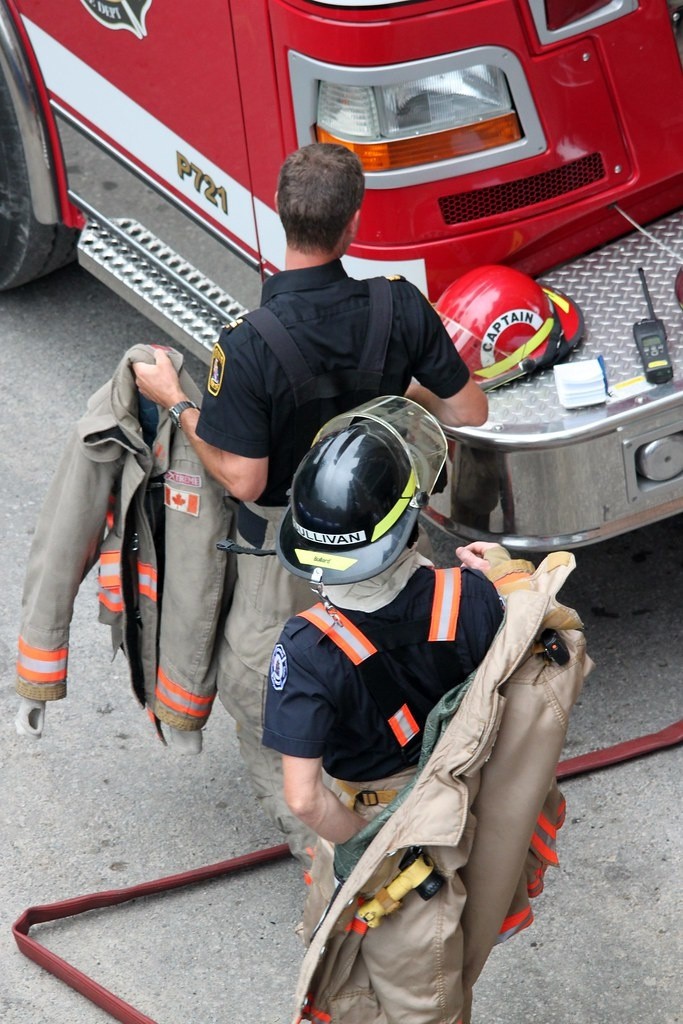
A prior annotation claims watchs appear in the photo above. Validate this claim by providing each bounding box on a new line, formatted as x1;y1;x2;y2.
168;400;203;429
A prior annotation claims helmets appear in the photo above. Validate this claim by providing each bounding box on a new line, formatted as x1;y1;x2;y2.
274;420;431;589
434;263;585;391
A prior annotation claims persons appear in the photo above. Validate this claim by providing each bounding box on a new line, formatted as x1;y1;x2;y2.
259;393;539;1023
130;145;492;947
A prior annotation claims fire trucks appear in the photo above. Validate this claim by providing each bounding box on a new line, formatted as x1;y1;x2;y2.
2;1;683;568
